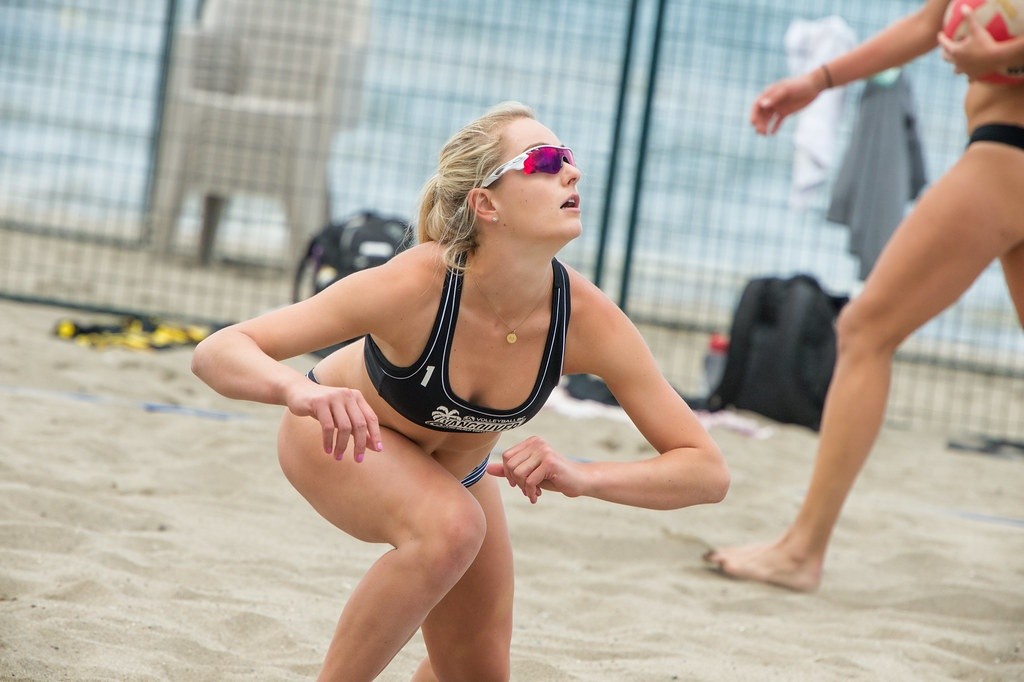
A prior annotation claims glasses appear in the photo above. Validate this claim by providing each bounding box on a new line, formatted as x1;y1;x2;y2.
483;145;575;187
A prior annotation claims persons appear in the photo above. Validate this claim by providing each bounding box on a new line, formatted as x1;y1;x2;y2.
697;0;1024;592
192;101;731;682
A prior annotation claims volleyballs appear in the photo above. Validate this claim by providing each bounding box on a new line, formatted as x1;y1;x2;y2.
940;0;1024;87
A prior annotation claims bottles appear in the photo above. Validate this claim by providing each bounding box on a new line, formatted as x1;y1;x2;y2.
704;337;729;393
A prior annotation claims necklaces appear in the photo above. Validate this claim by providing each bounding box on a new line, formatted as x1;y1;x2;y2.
467;252;552;345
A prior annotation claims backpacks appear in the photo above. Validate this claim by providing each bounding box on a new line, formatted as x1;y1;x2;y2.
708;273;851;433
296;208;414;359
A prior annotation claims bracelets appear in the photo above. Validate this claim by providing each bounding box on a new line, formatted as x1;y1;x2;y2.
821;64;834;87
